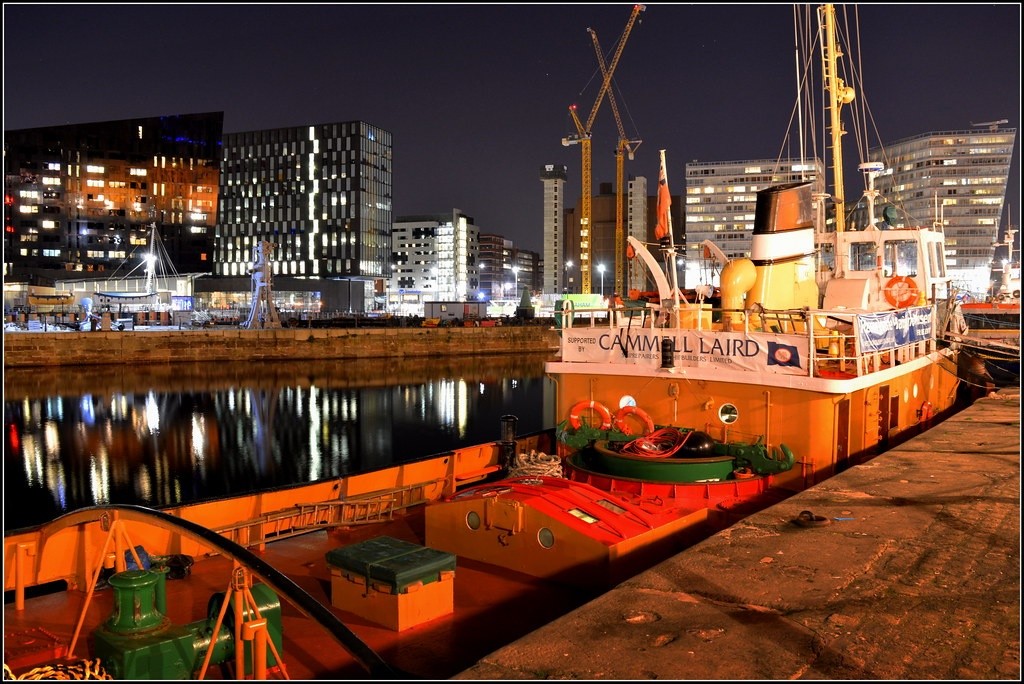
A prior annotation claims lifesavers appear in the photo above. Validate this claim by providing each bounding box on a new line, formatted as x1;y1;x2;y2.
615;405;656;437
568;400;612;431
883;275;919;308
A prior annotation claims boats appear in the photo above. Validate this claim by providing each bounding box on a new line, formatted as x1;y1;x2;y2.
27;294;75;305
4;3;961;684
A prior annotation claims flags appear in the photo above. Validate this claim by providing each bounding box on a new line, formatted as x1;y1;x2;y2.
654;150;672;240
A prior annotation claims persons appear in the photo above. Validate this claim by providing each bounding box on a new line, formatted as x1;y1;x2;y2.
260;313;270;329
210;315;218;325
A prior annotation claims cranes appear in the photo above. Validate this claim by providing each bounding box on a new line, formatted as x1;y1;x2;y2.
587;29;644;304
558;4;646;295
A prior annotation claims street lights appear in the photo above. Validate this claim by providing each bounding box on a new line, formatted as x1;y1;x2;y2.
677;259;683;288
566;261;572;298
512;266;519;299
478;264;485;296
597;264;605;306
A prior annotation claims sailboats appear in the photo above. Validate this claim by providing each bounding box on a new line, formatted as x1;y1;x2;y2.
93;222;207;305
